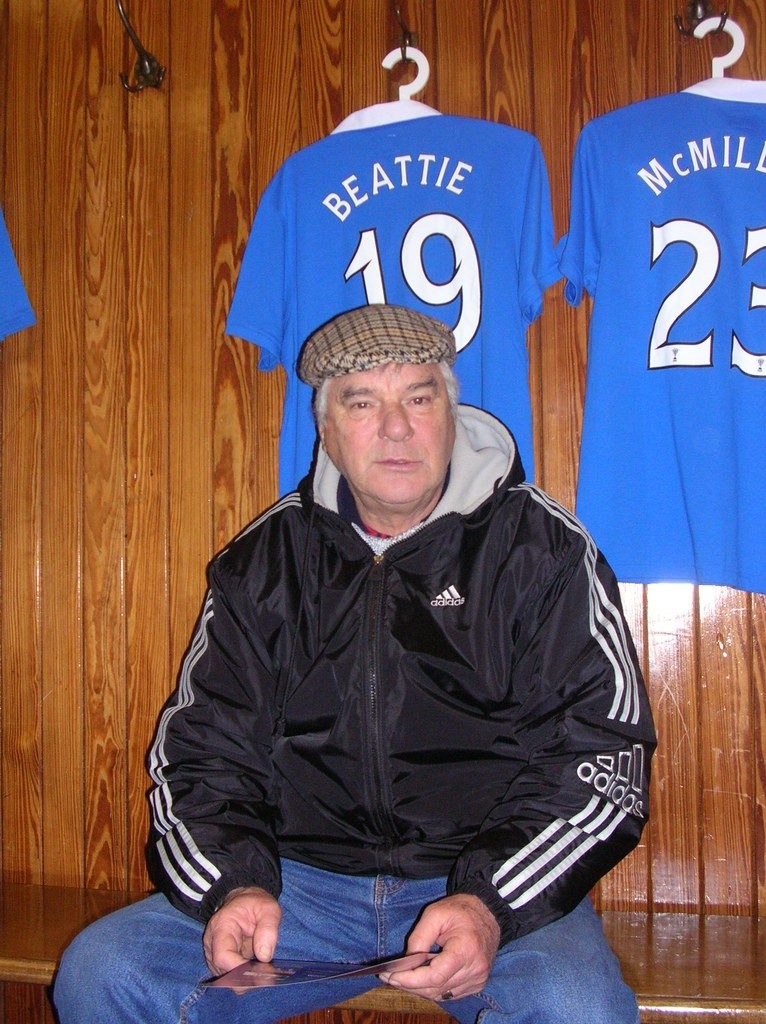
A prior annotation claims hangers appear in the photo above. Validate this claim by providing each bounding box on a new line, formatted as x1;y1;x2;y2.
693;17;746;77
380;46;431;100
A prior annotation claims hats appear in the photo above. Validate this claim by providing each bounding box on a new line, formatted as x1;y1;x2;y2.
298;305;456;388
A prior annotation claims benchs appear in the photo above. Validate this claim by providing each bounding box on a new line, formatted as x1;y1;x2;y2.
0;880;766;1024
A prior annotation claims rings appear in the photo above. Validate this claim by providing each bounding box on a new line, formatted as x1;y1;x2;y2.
441;989;453;1001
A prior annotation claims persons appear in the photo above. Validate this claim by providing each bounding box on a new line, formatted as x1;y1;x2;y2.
52;304;660;1024
